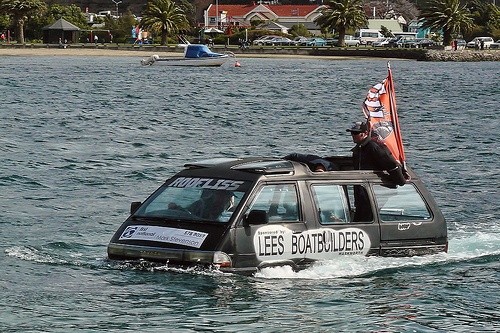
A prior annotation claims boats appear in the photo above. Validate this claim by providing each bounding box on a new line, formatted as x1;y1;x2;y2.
106;155;449;274
140;43;229;67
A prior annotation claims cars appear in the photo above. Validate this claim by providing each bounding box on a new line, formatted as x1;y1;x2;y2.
253;28;500;49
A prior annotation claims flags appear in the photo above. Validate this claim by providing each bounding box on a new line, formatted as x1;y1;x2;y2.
361;69;406;165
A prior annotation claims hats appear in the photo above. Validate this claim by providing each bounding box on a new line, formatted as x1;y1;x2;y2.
214;188;237;198
345;121;368;131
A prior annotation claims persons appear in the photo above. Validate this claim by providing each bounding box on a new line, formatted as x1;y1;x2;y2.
167;186;240;222
451;36;487;51
284;152;345;223
345;121;406;186
